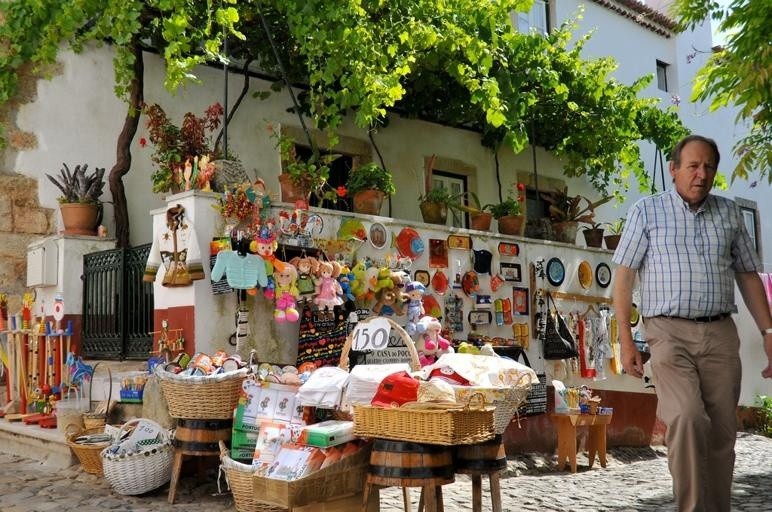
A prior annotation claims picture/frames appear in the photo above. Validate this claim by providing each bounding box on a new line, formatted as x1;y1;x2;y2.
473;289;495;309
466;308;493;326
367;221;390;250
445;235;473;252
627;301;641;327
305;215;326;238
414;268;432;288
511;285;531;318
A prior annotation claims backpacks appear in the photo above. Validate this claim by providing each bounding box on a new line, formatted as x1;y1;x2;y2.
217;438;290;512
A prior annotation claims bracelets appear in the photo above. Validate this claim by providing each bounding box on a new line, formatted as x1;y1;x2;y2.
760;327;772;336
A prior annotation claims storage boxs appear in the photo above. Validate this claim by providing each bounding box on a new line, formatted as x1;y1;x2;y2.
118;380;146;405
250;440;376;509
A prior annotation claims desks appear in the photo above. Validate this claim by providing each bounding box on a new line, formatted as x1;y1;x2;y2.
452;338;523;367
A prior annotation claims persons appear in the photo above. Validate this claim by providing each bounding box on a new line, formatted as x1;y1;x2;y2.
610;136;772;512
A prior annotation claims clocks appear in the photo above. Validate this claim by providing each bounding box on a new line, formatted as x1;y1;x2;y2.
592;261;611;288
575;259;594;292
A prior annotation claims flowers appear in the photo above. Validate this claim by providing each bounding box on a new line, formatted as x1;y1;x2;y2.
133;99;230;195
489;177;528;225
533;286;549;324
262;117;348;211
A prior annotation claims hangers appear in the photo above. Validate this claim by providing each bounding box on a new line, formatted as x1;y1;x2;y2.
232;235;250;260
578;298;601;322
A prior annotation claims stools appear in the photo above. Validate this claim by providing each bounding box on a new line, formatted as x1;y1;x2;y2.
549;407;613;473
164;418;240;505
361;438;456;512
418;434;510;512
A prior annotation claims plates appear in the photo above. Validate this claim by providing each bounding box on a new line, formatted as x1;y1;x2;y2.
446;234;472;250
462;271;481;299
467;286;530;349
578;260;594;291
472;248;494;275
596;262;612;288
630;302;640;327
389;228;426;261
368;221;388;248
415;270;447;296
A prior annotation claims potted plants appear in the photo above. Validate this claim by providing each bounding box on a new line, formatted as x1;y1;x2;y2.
538;184;624;253
42;161;109;237
447;187;495;231
341;159;394;218
415;184;454;226
206;143;246;194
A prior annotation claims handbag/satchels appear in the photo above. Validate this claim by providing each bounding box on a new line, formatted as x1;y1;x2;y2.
541;290;581;361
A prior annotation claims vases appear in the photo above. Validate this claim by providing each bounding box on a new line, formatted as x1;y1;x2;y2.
169;173;200;194
276;170;313;204
496;210;525;236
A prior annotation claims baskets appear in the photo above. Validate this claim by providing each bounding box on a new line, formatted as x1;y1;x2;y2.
65;421;133;476
327;316;424;423
350;390;500;449
100;418;185;499
154;358;250;420
482;372;535;435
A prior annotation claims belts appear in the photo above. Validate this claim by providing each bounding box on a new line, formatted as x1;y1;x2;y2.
655;310;733;325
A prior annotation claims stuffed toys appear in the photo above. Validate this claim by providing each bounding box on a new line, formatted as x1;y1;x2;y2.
210;179;454;369
